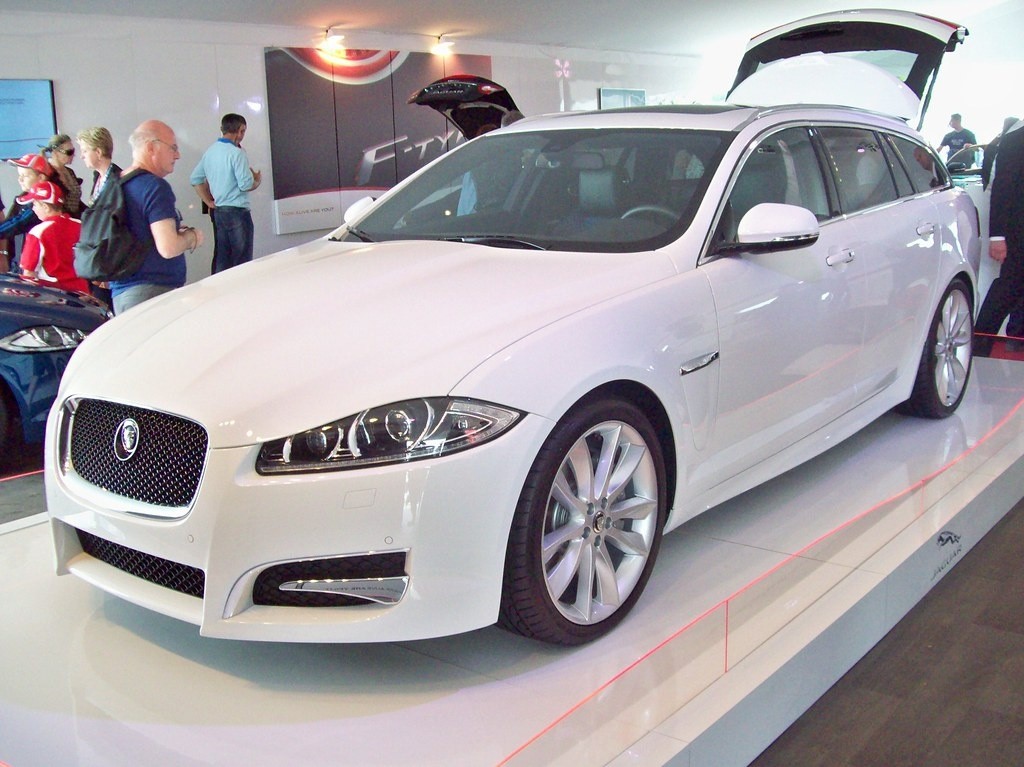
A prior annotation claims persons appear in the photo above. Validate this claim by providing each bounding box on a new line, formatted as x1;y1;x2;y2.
453;111;530;214
936;115;1024;359
0;113;262;316
855;143;947;209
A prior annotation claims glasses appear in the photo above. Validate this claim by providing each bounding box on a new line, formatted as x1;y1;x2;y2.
152;139;178;154
56;147;75;156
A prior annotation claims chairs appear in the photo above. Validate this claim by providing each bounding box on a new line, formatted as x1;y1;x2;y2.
723;143;789;230
567;153;632;226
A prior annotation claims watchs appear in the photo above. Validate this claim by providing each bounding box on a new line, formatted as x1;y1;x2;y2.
0;250;9;256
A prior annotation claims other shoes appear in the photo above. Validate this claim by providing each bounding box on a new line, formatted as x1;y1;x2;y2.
1006;342;1024;351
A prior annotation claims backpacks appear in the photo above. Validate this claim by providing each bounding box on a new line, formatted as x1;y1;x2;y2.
72;167;153;282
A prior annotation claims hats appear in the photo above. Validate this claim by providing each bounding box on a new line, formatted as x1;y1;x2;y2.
15;180;66;206
7;154;55;178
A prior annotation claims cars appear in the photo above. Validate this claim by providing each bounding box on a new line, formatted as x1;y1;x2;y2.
0;268;117;474
944;144;990;234
43;10;979;649
